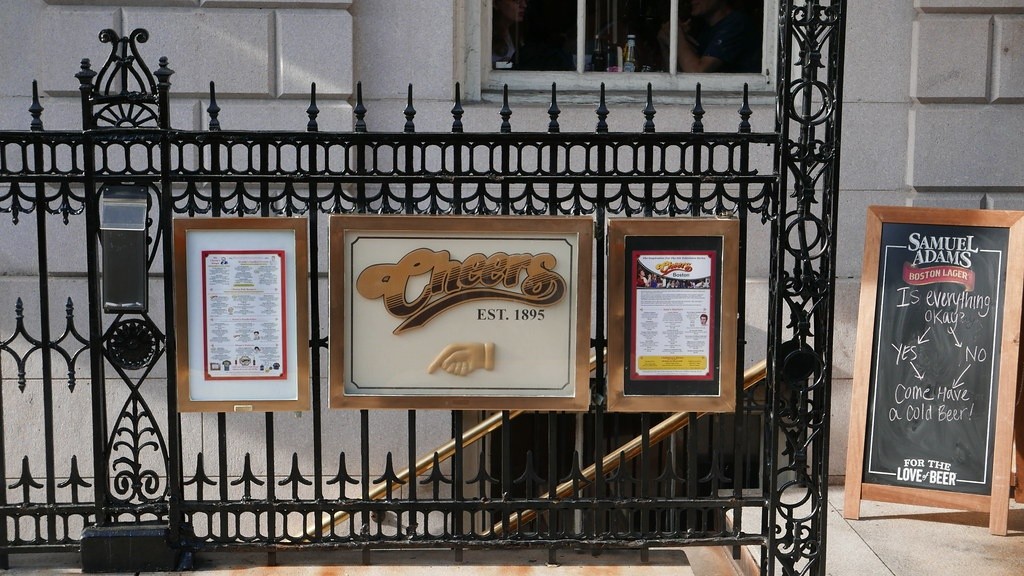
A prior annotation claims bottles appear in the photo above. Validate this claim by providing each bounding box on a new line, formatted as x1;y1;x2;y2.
622;35;640;72
591;34;605;71
606;39;615;72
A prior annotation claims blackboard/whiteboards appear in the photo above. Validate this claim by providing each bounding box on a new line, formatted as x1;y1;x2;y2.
858;209;1013;500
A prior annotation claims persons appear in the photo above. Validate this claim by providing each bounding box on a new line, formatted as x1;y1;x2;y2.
221;257;228;264
253;331;260;340
700;314;708;326
638;268;695;289
254;347;259;353
